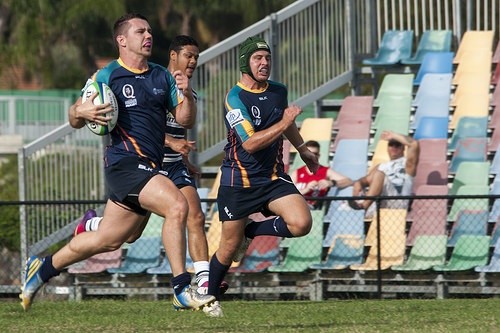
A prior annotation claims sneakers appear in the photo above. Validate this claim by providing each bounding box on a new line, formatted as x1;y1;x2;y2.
203;301;221;316
233;217;254;262
170;273;215;311
19;255;46;311
75;209;97;236
192;274;228;297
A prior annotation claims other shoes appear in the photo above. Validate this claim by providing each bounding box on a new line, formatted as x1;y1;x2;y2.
348;199;363;210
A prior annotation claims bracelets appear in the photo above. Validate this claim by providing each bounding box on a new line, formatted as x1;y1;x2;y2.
295;141;305;150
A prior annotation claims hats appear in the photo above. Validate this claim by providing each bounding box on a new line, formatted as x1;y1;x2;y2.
239;37;272;72
389;139;402;147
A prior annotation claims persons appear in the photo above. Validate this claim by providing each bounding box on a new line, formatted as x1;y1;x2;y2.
347;129;422;217
74;35;225;318
18;13;216;311
289;139;354;209
204;35;319;317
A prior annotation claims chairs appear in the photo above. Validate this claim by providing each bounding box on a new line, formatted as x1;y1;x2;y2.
66;29;500;299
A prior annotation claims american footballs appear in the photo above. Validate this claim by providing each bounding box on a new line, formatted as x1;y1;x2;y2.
79;82;118;135
